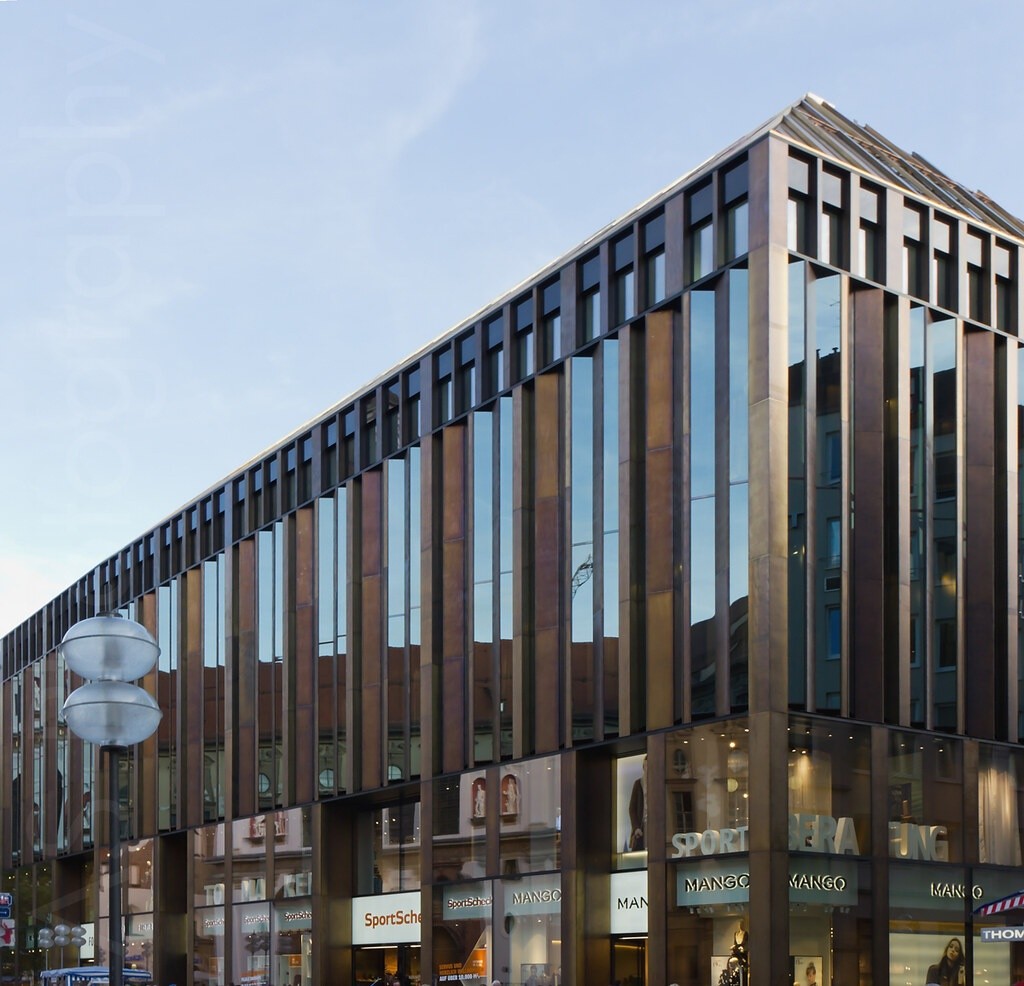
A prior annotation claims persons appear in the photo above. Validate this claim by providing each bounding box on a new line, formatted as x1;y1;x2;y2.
525;966;538;986
629;755;647;851
475;785;485;815
806;962;816;986
730;919;748;954
480;980;500;986
503;778;516;812
926;938;966;986
371;973;399;986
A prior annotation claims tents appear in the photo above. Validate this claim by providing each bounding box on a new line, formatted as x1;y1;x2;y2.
40;966;151;986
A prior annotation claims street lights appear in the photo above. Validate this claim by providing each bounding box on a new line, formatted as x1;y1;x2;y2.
260;931;269;979
58;609;166;985
54;924;71;968
140;942;152;972
38;927;54;972
242;934;261;977
70;926;87;968
